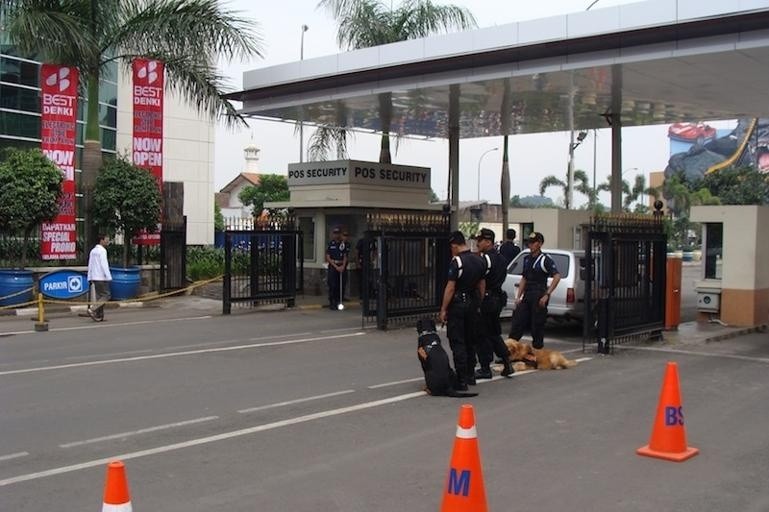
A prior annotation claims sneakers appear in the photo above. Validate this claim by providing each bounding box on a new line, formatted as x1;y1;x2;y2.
86;304;103;321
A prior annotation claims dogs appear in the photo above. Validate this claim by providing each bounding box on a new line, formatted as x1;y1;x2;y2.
393;283;428;301
416;319;479;397
493;338;579;372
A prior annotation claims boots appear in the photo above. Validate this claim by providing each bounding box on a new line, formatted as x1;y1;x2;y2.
451;363;476;392
475;360;492;379
500;355;514;376
328;296;339;311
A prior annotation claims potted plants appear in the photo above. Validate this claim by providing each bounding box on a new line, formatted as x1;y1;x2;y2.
0;143;68;316
86;149;164;300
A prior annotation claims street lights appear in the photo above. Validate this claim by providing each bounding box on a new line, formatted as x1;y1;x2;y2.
564;132;587;208
622;167;638;176
477;147;499;201
300;22;310;163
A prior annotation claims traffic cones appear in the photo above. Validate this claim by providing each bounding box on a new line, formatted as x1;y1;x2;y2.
636;360;701;463
438;400;489;512
101;460;133;511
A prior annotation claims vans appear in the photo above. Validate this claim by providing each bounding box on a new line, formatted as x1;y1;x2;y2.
501;248;654;333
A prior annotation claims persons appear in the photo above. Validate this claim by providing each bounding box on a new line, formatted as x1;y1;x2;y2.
469;226;515;379
497;229;521;265
494;231;561;364
324;226;347;312
353;230;378;304
86;233;115;323
436;230;488;391
341;232;351;303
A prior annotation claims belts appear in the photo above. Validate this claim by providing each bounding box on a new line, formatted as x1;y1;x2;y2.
331;259;343;264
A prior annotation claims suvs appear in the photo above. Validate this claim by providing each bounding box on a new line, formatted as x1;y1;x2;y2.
673;229;700;251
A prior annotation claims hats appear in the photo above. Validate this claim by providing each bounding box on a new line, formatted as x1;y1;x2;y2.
525;231;544;243
447;231;462;244
507;229;516;235
330;225;349;237
470;228;495;242
